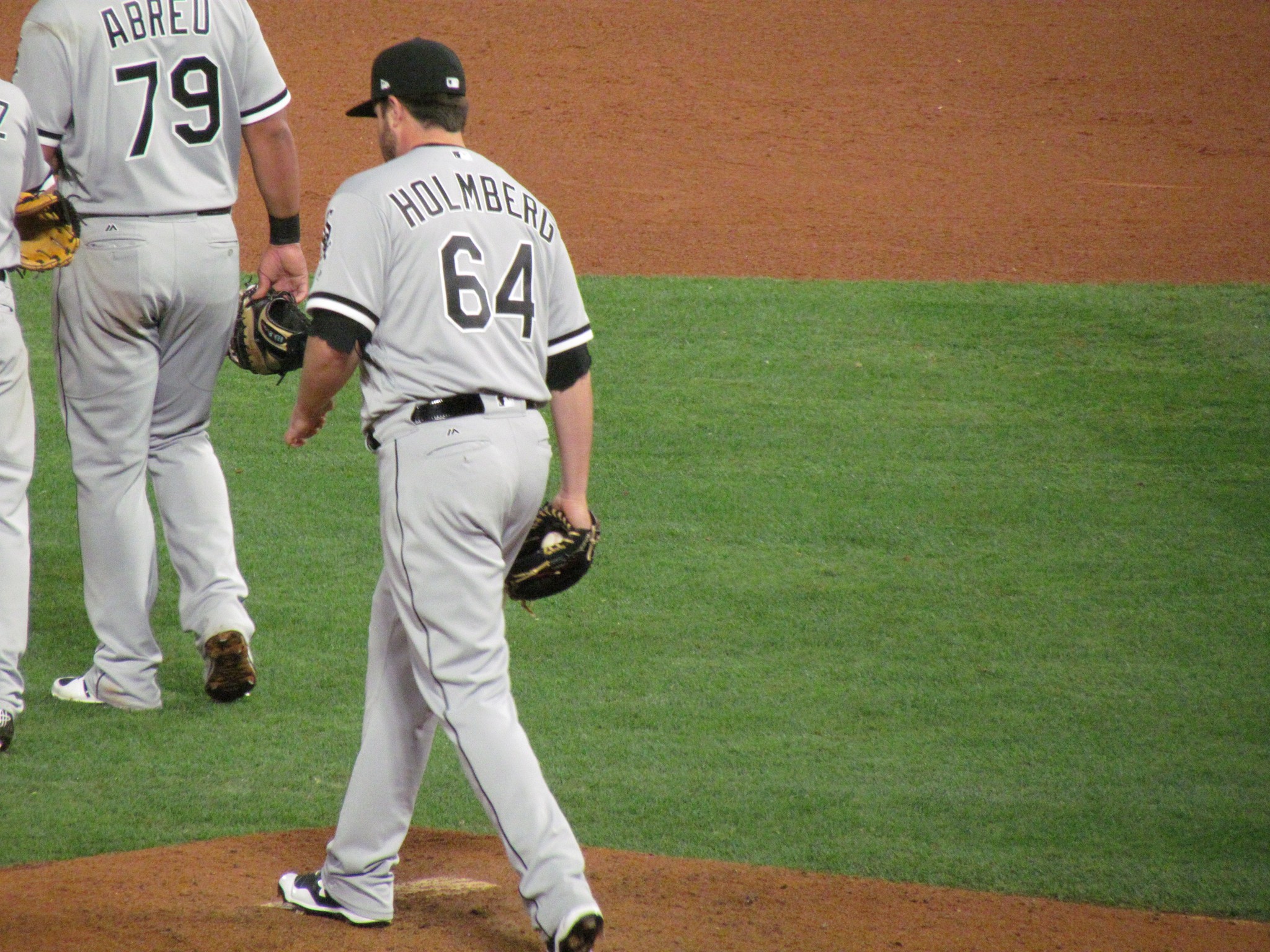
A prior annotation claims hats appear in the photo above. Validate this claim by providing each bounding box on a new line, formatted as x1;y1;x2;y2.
344;38;466;118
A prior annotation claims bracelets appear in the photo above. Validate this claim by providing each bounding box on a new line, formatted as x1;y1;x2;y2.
268;214;300;246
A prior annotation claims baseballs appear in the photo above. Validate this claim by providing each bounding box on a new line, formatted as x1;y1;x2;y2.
541;532;564;549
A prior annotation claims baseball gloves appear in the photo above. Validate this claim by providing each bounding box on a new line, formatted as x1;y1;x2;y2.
505;502;601;602
227;284;312;376
13;191;81;273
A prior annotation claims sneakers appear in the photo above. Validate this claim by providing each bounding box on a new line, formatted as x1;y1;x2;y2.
0;710;14;751
277;870;392;928
51;676;105;704
545;903;606;952
201;631;256;703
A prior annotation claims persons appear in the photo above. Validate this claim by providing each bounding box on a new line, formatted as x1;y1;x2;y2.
13;0;308;710
277;37;607;952
0;77;80;762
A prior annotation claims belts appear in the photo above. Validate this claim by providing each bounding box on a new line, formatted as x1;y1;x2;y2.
0;269;6;282
368;392;537;451
197;205;232;216
